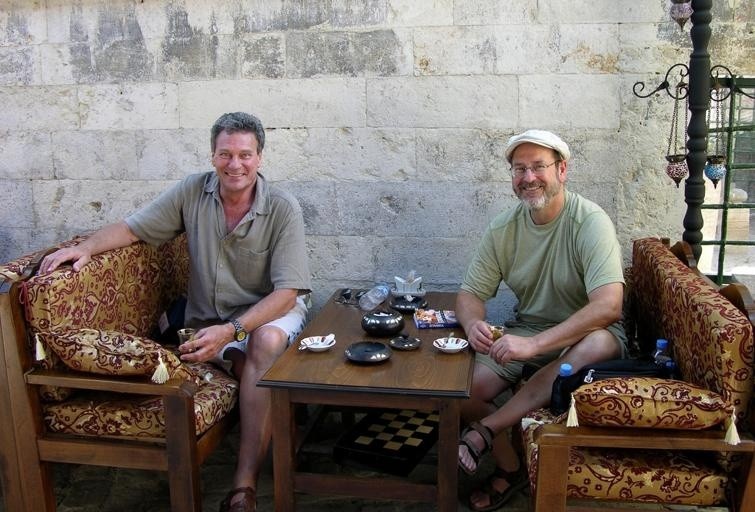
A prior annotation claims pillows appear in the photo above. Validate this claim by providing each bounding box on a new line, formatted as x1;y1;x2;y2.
566;379;745;447
33;326;213;390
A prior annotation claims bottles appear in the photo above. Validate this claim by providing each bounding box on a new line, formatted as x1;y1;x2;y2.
651;339;671;368
358;283;391;312
550;362;575;417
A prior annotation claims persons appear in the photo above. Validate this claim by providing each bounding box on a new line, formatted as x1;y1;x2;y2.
454;129;630;512
37;113;313;512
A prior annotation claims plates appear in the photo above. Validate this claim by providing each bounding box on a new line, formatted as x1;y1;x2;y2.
346;340;392;364
433;337;469;354
387;297;429;312
301;335;336;352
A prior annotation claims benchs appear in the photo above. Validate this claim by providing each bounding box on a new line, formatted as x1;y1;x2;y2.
1;225;242;510
508;237;755;511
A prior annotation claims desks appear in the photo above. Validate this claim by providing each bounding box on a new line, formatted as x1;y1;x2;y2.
258;286;481;512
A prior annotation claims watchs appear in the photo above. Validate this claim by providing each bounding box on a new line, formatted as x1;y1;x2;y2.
229;318;246;343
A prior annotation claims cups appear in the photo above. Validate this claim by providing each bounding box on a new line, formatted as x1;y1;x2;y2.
489;326;505;342
178;328;196;358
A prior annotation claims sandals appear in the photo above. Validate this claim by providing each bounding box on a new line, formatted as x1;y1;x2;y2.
219;487;258;512
469;462;529;510
458;421;493;475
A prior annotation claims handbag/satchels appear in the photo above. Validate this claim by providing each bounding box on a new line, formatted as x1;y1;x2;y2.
552;359;675;415
147;294;187;341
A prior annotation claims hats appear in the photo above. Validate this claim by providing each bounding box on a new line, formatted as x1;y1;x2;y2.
505;130;570;163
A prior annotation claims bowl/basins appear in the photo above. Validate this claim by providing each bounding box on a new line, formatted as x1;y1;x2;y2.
361;309;405;338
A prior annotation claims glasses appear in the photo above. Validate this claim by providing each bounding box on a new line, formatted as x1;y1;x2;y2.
333;296;359;307
508;159;562;177
